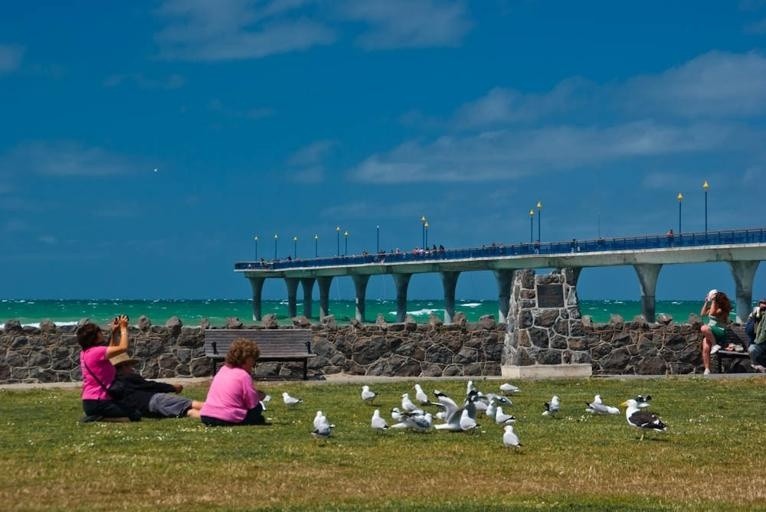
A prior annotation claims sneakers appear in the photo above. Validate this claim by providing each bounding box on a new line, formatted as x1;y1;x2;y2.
704;367;710;375
709;344;721;354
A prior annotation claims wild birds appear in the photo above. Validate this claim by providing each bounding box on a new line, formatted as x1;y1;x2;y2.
585;394;652;415
309;410;335;447
390;381;523;434
618;399;668;442
281;392;302;410
371;409;390;436
502;424;524;454
361;385;378;404
542;394;561;419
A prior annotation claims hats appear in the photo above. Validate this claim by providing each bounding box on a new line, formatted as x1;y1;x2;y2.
110;352;139;366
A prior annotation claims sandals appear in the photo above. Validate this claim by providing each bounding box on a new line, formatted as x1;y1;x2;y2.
81;414;103;422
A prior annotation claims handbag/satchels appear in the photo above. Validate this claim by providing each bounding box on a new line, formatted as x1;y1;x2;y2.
108;380;137;408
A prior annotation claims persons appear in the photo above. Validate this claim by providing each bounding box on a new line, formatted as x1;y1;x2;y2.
109;352;204;420
363;238;576;263
667;229;674;247
700;289;732;374
76;314;142;425
744;300;766;372
199;338;267;426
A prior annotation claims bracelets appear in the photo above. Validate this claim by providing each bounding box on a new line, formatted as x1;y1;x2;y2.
120;332;129;336
